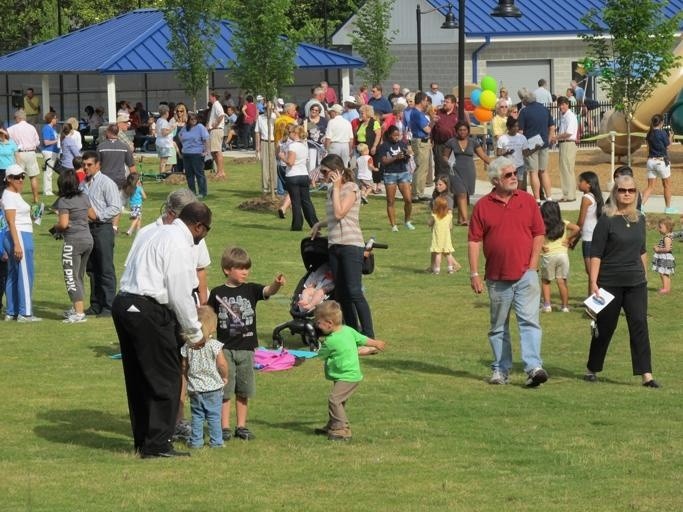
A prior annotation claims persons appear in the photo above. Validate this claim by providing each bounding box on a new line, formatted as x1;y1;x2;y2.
0;80;682;457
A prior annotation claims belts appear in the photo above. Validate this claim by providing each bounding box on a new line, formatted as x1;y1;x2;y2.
261;138;274;142
117;290;149;301
19;149;35;152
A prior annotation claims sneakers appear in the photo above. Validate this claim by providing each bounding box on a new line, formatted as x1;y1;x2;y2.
234;425;256;440
405;220;415;230
541;302;552;313
126;230;131;235
172;420;205;440
222;428;233;441
557;199;576;202
643;380;664;388
489;370;509;385
392;225;399;232
314;426;351;440
61;304;112;324
665;207;679;214
4;314;16;321
641;204;647;212
361;188;385;204
43;191;55;195
557;305;569;313
584;375;596;381
525;367;548;387
16;313;42;321
424;262;461;274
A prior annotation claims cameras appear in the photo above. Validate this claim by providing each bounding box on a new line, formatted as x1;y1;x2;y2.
400;149;411;164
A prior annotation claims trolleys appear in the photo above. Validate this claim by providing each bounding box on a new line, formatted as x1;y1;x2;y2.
42;157;74;176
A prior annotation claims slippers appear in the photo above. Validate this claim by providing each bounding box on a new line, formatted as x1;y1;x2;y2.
278;209;286;219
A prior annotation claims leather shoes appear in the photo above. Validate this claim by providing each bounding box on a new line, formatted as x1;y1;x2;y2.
135;446;191;459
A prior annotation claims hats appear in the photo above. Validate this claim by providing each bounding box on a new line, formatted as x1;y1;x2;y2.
326;104;344;114
3;164;26;181
341;96;357;104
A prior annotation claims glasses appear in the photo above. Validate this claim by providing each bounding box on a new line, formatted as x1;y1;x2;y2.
505;170;517;178
8;175;25;180
617;188;637;194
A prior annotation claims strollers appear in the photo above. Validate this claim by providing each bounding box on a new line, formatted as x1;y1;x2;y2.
270;219;389;354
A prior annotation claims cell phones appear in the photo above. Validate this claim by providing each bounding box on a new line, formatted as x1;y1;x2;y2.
328;171;343;183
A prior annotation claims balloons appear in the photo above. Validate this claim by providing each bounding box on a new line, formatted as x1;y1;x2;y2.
480;76;497;95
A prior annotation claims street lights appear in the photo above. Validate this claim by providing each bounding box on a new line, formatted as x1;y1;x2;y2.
416;2;458;91
54;0;75;125
458;0;524;126
